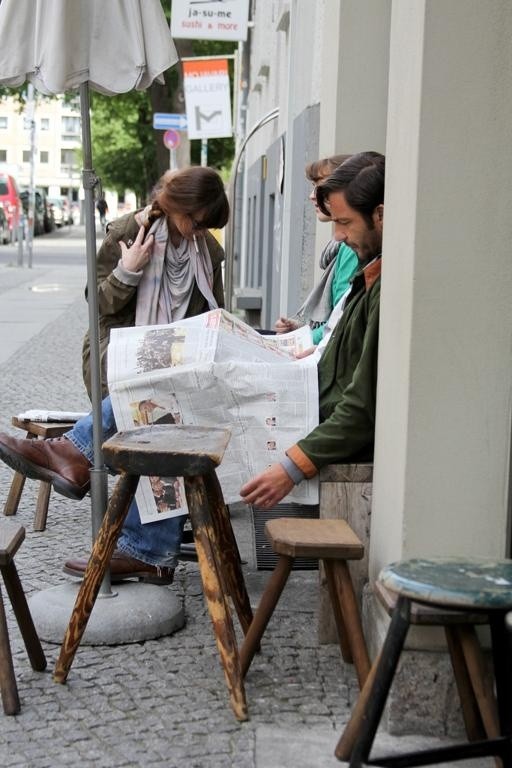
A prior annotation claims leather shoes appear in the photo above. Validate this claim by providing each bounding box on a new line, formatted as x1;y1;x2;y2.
60;547;175;586
0;431;94;502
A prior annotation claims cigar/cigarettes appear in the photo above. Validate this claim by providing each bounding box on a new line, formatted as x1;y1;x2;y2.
193;233;201;254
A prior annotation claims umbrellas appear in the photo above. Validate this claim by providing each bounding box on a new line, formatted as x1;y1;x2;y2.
1;1;183;599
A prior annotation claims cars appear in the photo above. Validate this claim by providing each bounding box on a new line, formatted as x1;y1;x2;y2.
0;172;80;246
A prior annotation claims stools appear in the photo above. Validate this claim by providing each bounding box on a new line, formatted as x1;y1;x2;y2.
4;415;76;531
0;523;47;716
239;518;372;692
54;425;260;721
335;555;511;767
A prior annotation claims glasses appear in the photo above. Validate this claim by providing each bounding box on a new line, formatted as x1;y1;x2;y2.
186;211;205;231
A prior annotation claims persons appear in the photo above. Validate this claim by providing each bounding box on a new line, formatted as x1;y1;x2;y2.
1;150;388;584
254;152;359;347
78;165;232;408
131;396;186;511
95;200;109;226
